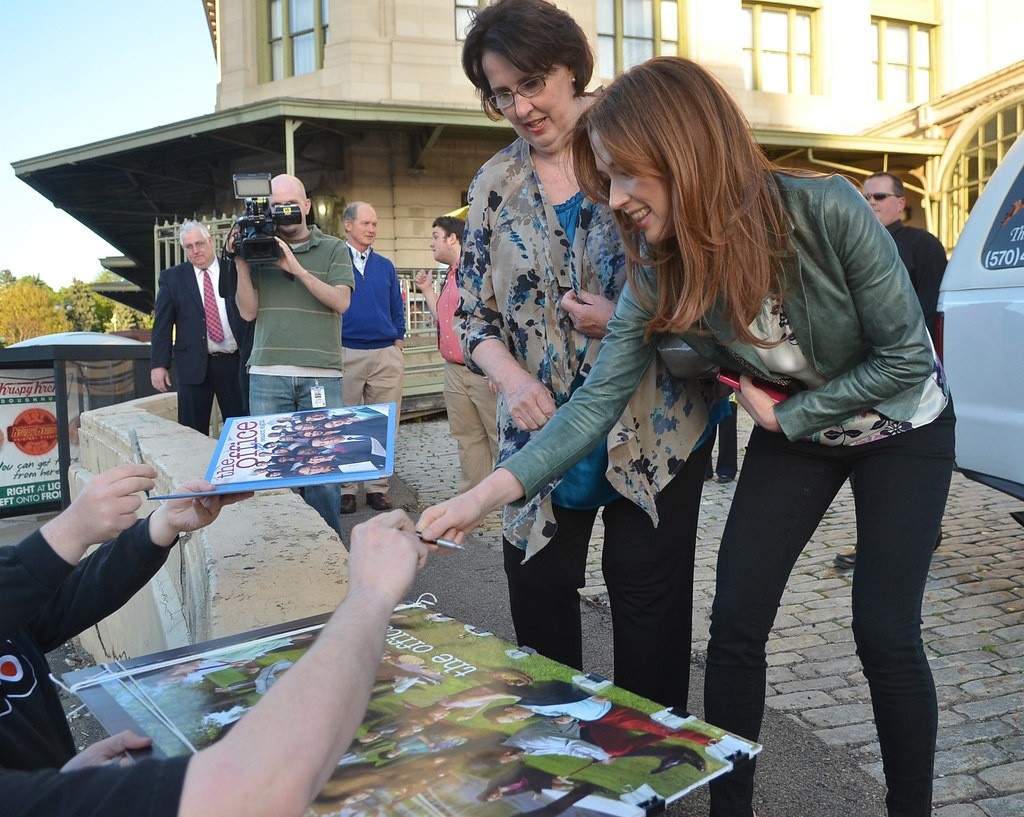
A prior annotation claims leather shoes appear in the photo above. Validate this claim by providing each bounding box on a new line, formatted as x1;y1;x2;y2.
339;493;356;514
365;491;392;510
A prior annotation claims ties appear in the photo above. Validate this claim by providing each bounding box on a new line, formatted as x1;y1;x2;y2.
204;267;225;345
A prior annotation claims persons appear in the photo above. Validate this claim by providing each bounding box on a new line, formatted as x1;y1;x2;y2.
1;1;947;817
143;611;719;817
253;406;386;479
415;56;956;817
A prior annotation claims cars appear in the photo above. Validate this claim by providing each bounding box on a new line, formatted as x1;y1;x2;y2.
935;128;1024;523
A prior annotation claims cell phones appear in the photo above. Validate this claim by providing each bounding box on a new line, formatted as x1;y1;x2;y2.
716;367;788;402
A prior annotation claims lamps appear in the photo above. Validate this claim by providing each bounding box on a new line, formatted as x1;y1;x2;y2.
307;173;339;236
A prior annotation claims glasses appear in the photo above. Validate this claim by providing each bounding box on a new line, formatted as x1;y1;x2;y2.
489;67;553;108
183;239;209;249
860;191;895;202
